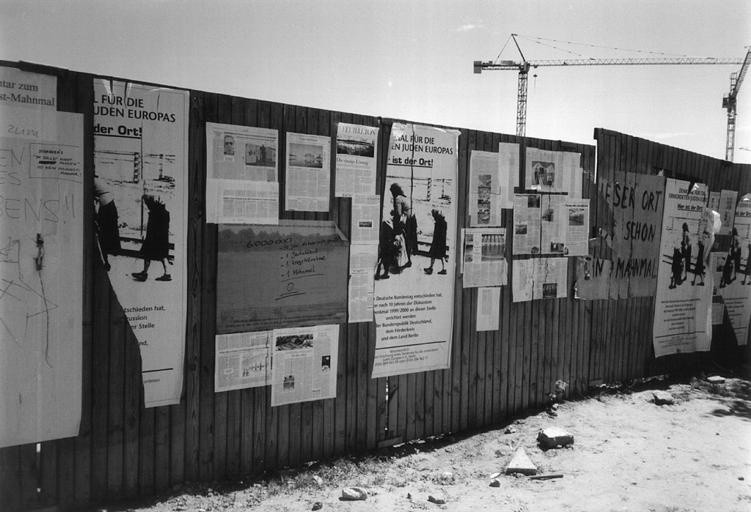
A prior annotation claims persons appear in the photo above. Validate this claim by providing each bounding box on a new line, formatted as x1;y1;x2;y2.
375;181;449;279
130;178;174;282
667;221;751;289
92;176;124;273
222;134;236;154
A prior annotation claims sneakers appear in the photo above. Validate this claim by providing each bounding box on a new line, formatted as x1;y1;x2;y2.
438;269;447;274
424;267;433;274
691;280;704;286
375;273;390;280
155;274;172;281
669;285;677;289
132;271;148;282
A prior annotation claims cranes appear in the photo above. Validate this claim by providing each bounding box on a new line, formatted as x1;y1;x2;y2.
723;47;751;165
474;31;750;138
